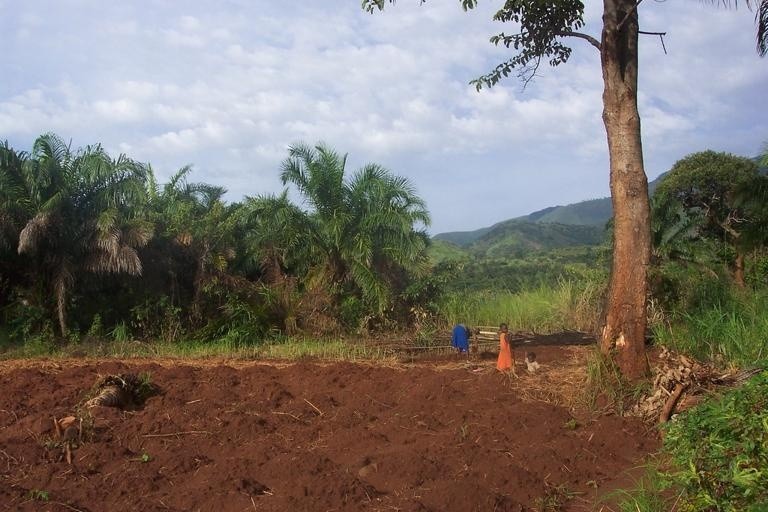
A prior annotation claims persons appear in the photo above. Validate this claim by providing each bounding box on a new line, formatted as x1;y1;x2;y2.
525;352;541;375
453;324;483;357
497;321;516;372
